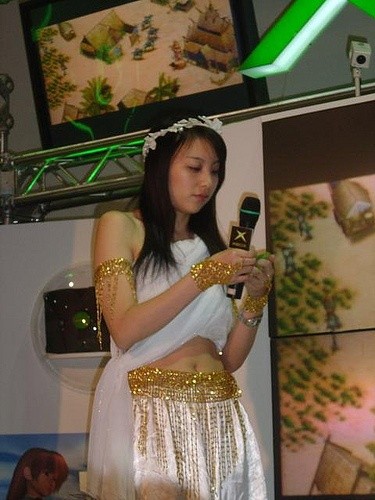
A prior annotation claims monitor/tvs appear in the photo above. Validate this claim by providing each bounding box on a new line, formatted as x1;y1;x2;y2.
18;0;271;150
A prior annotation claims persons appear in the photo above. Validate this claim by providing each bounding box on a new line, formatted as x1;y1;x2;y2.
86;116;274;500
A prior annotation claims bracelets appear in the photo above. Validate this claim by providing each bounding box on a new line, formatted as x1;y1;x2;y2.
190;258;243;292
243;285;271;312
240;311;263;327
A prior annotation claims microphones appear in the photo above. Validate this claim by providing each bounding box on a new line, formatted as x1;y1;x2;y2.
226;197;261;299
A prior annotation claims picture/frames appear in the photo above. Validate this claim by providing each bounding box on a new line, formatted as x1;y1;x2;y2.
19;0;271;150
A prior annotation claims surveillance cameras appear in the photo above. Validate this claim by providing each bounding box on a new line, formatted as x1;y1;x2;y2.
348;40;371;68
